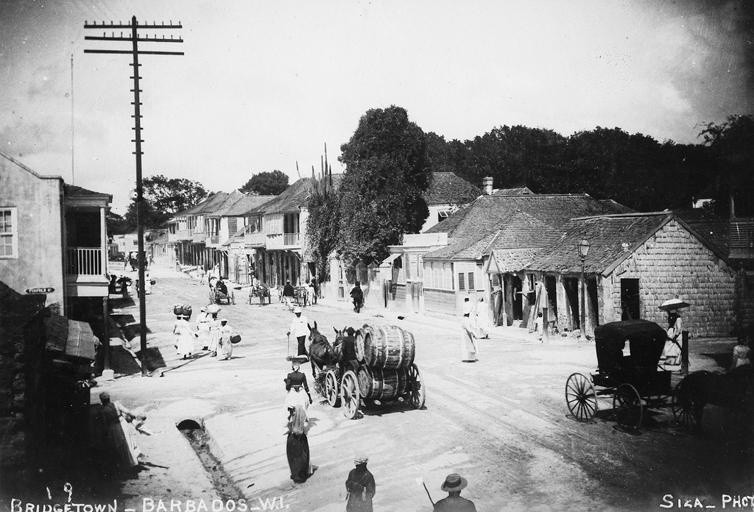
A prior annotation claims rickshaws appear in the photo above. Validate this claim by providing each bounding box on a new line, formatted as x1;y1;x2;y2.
278;285;308;305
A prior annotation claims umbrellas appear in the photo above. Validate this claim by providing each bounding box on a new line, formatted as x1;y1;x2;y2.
658;297;689;315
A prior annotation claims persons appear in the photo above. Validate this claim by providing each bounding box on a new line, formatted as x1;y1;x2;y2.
533;311;544;343
287;404;314;483
169;300;237;362
461;296;477;339
283;362;313;423
474;294;491;340
431;474;477;512
459;312;479;361
120;250;155;297
286;307;309;364
349;281;363;314
338;325;355;384
664;308;683;365
214;275;319;312
345;454;375;512
667;313;676;329
730;335;751;370
282;378;311;437
93;390;141;472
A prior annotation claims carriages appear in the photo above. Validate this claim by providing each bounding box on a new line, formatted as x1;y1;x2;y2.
304;321;426;419
108;244;125;261
249;275;271;305
129;250;149;270
205;271;235;306
565;318;753;434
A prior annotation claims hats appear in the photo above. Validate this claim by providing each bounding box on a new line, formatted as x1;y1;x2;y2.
354;453;369;465
440;473;468;492
293;307;302;314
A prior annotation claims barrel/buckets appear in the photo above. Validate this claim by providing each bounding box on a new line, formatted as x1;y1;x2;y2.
353;326;415;400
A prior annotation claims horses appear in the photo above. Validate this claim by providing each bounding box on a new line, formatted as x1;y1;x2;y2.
333;327;347;364
308;321;333;378
